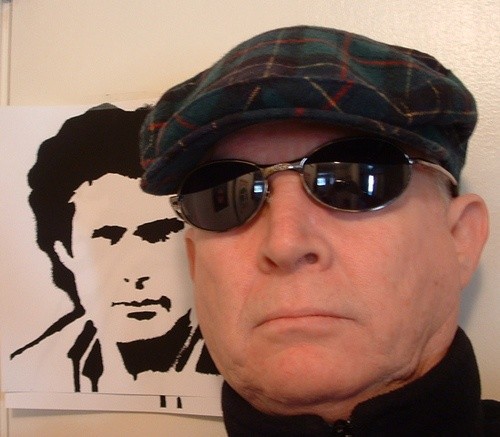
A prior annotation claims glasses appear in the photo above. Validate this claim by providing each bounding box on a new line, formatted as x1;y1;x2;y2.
168;131;461;233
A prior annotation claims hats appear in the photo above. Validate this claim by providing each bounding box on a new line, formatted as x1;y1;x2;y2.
134;25;478;194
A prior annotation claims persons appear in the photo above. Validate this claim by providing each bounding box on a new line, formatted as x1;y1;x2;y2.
136;25;499;436
